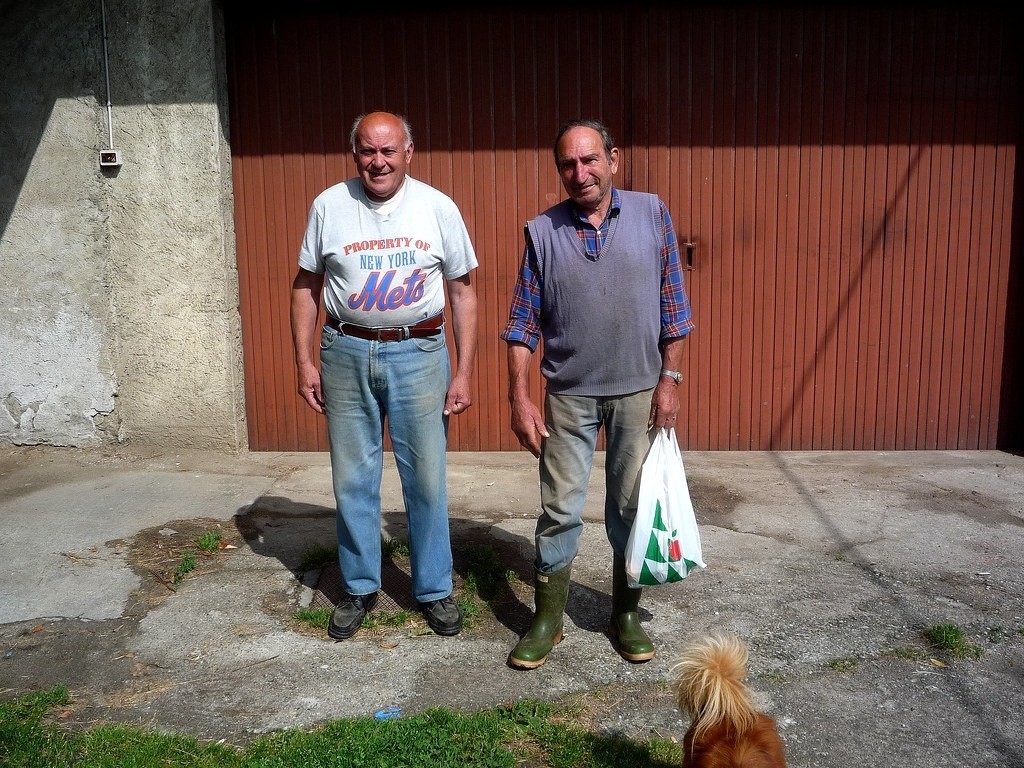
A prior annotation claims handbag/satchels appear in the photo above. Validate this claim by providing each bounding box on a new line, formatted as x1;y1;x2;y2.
624;424;708;587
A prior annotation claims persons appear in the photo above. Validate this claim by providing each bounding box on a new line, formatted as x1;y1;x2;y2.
289;112;478;639
497;121;696;668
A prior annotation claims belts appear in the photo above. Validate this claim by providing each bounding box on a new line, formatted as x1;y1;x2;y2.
325;312;444;341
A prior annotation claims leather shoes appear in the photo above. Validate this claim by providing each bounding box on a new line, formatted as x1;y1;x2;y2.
327;593;375;639
421;597;463;635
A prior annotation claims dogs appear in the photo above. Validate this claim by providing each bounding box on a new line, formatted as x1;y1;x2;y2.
668;630;789;768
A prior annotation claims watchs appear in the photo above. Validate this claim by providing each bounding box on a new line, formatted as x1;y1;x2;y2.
662;370;684;384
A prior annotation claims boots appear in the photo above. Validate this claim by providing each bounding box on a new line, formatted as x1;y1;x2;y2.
608;556;655;663
508;563;571;668
